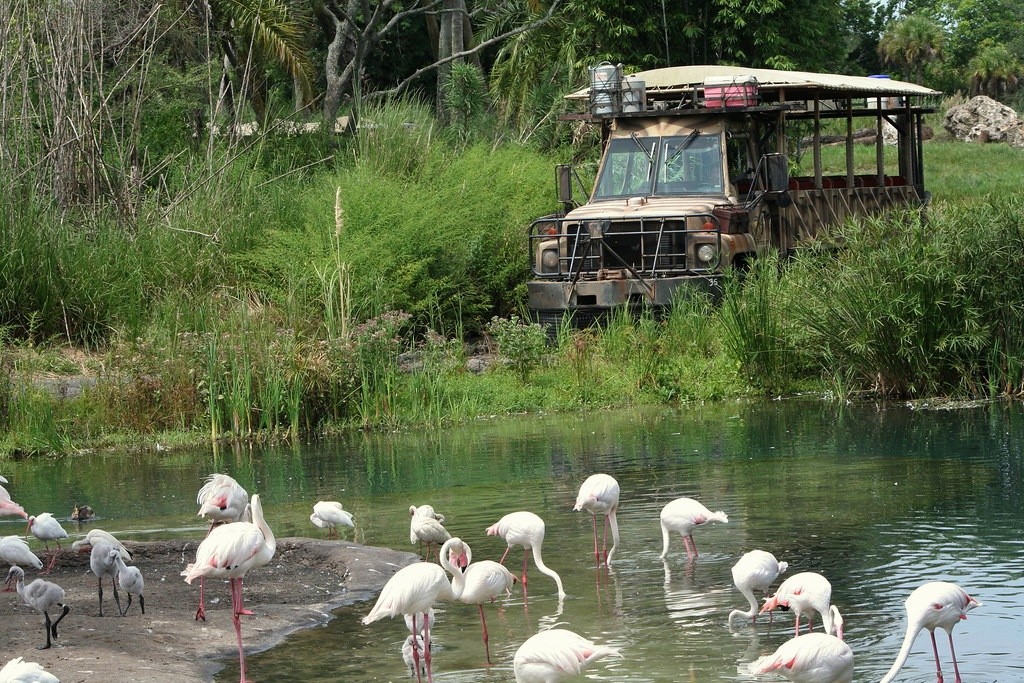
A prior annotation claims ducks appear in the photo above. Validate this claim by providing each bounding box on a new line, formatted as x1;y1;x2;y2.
71;502;95;521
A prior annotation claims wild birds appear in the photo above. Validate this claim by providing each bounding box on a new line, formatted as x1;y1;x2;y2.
26;512;68;575
197;472;252;539
409;504;452;566
761;572;843;641
0;475;28;519
879;582;980;683
310;501;354;539
486;510;566;596
5;566;70;651
0;656;60;683
180;493;276;683
362;536;518;683
72;528;145;617
0;535;43;592
513;629;625;683
573;474;620;565
748;632;854;683
728;549;788;629
659;497;729;558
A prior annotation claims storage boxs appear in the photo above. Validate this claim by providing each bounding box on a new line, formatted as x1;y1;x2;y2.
703;75;758;106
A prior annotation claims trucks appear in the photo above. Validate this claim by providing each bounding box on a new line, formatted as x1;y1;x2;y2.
527;61;942;345
